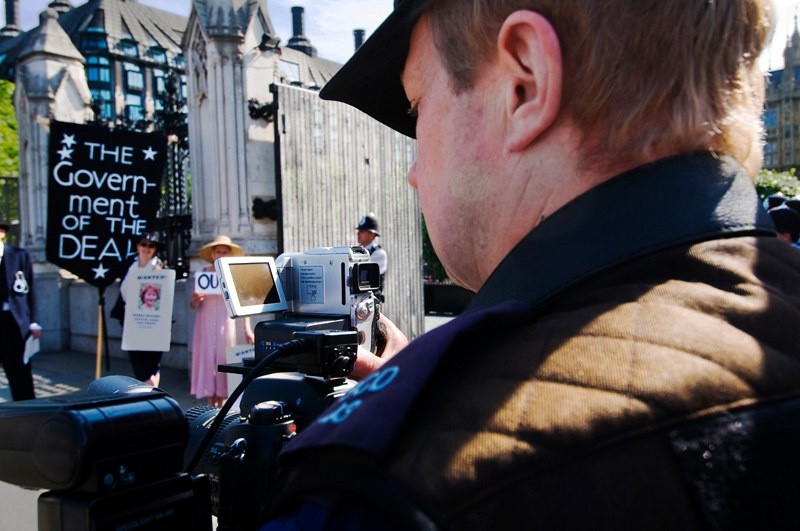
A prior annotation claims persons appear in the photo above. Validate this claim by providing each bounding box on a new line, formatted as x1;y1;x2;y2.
761;191;800;252
355;213;387;293
111;231;174;388
140;284;160;312
220;0;800;531
189;236;254;408
0;211;42;402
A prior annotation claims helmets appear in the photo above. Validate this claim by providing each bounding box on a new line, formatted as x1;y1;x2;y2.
0;211;11;232
356;216;381;235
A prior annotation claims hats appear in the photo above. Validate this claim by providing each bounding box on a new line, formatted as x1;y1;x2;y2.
197;235;244;262
319;0;423;140
138;232;159;242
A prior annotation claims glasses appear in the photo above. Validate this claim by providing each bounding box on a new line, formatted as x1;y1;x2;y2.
140;242;156;247
